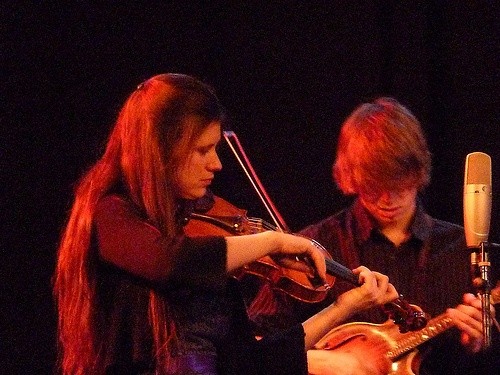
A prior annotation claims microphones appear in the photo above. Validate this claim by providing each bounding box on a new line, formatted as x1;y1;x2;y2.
463;152;492;284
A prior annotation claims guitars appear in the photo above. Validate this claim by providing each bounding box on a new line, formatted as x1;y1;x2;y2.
304;287;500;375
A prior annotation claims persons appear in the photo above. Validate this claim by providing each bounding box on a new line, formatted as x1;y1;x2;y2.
247;98;500;375
53;73;400;375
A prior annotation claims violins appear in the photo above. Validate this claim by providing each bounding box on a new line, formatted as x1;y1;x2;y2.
178;192;427;332
